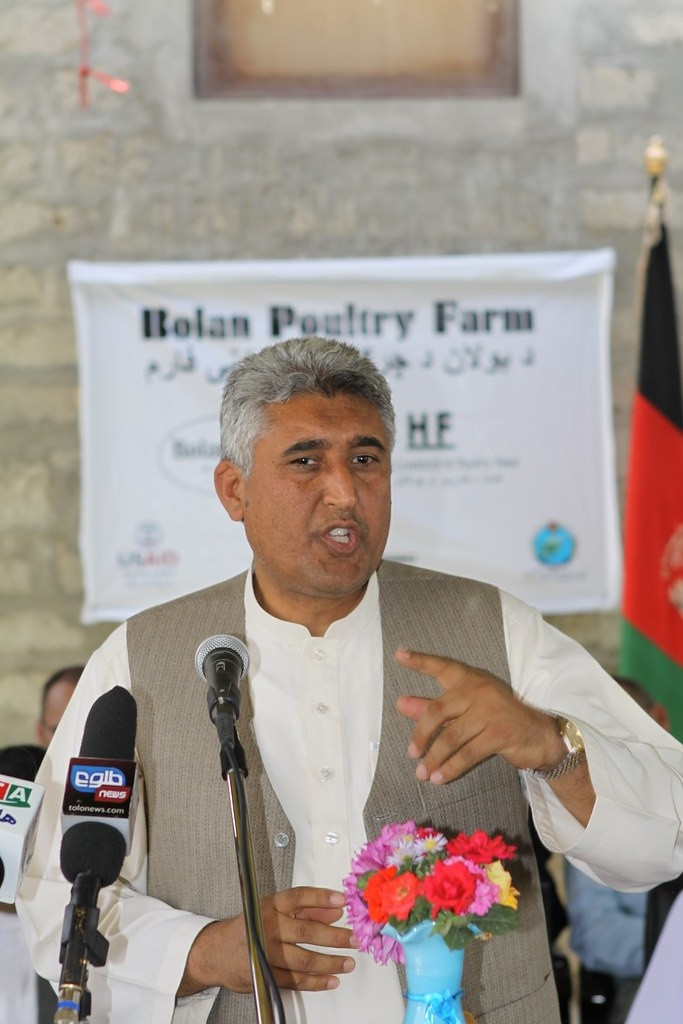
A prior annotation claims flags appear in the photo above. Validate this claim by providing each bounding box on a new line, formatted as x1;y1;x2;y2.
617;203;683;746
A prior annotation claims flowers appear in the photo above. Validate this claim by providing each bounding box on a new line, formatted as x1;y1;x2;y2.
340;819;524;968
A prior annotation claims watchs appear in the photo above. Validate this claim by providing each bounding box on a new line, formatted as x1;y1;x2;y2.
526;711;587;782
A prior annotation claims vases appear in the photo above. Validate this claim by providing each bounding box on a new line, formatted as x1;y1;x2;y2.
380;921;481;1024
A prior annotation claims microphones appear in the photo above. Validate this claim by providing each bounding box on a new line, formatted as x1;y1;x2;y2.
0;775;44;903
57;686;141;991
195;633;250;750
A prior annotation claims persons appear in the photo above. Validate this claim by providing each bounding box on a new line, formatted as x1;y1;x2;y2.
35;665;87;750
15;337;683;1024
562;678;671;1024
